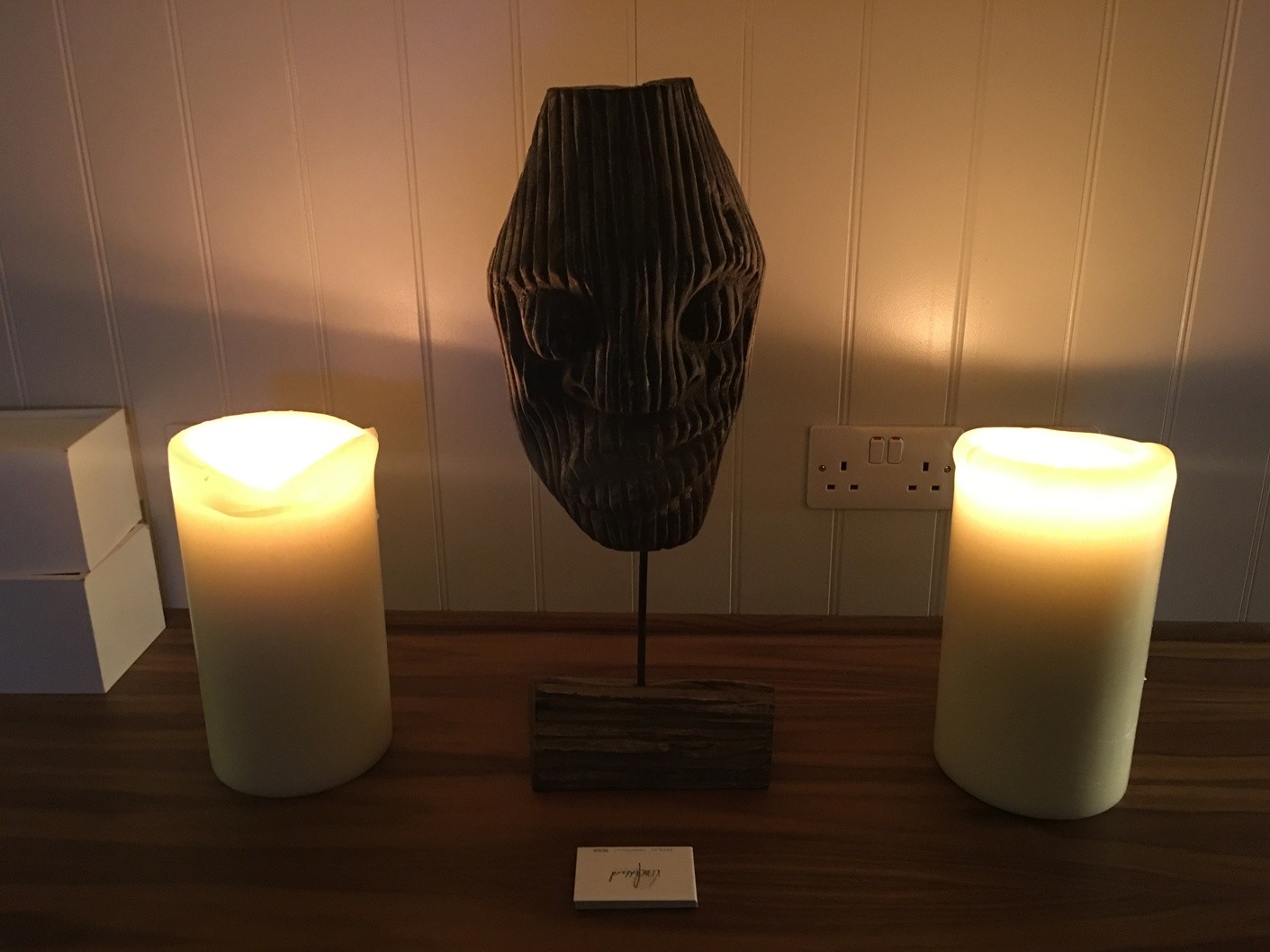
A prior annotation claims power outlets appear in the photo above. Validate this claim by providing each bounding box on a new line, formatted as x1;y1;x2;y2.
806;423;967;512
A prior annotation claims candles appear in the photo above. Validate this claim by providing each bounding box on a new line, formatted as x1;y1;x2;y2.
932;425;1179;822
167;409;394;798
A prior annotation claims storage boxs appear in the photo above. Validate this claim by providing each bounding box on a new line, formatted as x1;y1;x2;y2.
0;408;144;578
0;522;169;696
